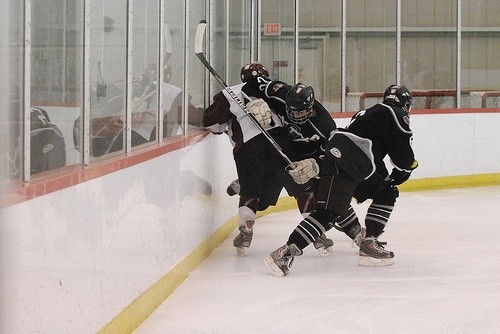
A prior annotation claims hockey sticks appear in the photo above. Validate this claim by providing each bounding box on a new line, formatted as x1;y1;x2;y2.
193;19;297;171
222;128;345;233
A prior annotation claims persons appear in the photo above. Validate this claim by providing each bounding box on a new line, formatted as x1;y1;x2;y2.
202;61;335;250
226;75;367;244
269;85;420;277
8;62;206;180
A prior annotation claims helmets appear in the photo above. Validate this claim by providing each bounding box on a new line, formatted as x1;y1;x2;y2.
284;82;315;125
382;84;414;114
240;60;272;83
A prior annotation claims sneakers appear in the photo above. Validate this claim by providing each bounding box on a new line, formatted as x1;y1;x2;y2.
357;235;396;267
263;243;303;278
312;234;336;259
232;217;256;258
350;224;366;247
226;178;241;197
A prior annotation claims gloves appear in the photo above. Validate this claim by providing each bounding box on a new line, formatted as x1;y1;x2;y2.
244;96;274;127
283;158;321;184
386;158;420;187
